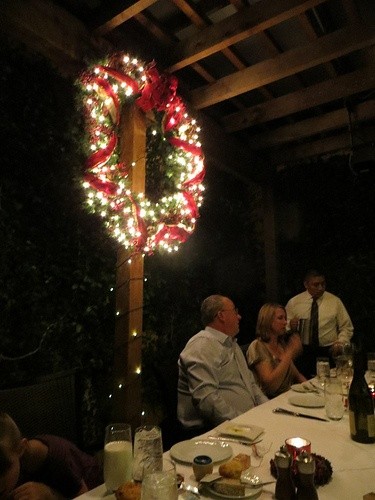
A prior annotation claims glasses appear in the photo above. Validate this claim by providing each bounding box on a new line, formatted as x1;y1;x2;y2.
222;307;238;312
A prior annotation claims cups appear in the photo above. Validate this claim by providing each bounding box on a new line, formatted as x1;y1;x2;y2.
103;423;133;493
332;341;353;392
316;357;330;387
140;458;178;500
134;425;163;483
323;378;344;420
193;455;213;482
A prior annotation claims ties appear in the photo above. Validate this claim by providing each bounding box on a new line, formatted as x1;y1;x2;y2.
309;296;320;358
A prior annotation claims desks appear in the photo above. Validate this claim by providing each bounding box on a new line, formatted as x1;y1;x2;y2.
74;368;375;500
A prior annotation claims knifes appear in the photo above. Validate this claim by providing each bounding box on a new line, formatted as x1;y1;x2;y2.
273;408;329;422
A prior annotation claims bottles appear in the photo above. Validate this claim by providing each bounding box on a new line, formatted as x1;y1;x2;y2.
363;360;375;401
347;352;375;444
273;449;319;500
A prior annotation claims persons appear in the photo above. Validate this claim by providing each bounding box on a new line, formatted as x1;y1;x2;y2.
0;411;105;500
246;302;307;400
175;295;269;441
285;272;354;379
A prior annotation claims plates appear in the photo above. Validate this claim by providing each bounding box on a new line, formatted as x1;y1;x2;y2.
169;438;233;464
214;420;264;442
288;396;326;408
290;384;318;392
204;470;263;499
